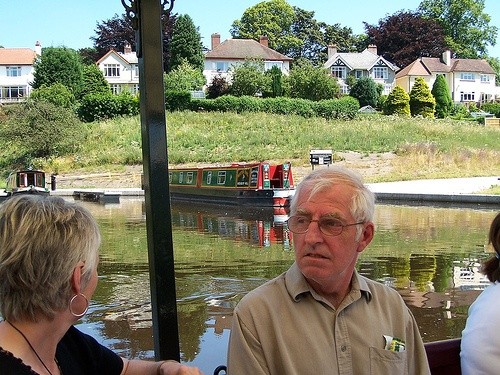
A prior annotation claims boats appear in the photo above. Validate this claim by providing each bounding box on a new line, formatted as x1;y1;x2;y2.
142;192;293;252
141;160;296;199
8;168;52;197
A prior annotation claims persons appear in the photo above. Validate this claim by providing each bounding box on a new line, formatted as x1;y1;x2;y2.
226;165;432;375
0;193;205;375
460;212;500;375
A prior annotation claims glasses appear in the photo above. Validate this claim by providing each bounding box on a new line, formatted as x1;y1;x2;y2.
285;214;364;236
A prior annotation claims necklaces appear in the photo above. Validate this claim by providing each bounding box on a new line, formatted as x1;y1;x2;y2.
4;316;61;375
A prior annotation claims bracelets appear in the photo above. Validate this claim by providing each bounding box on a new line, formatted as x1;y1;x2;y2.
157;359;180;375
